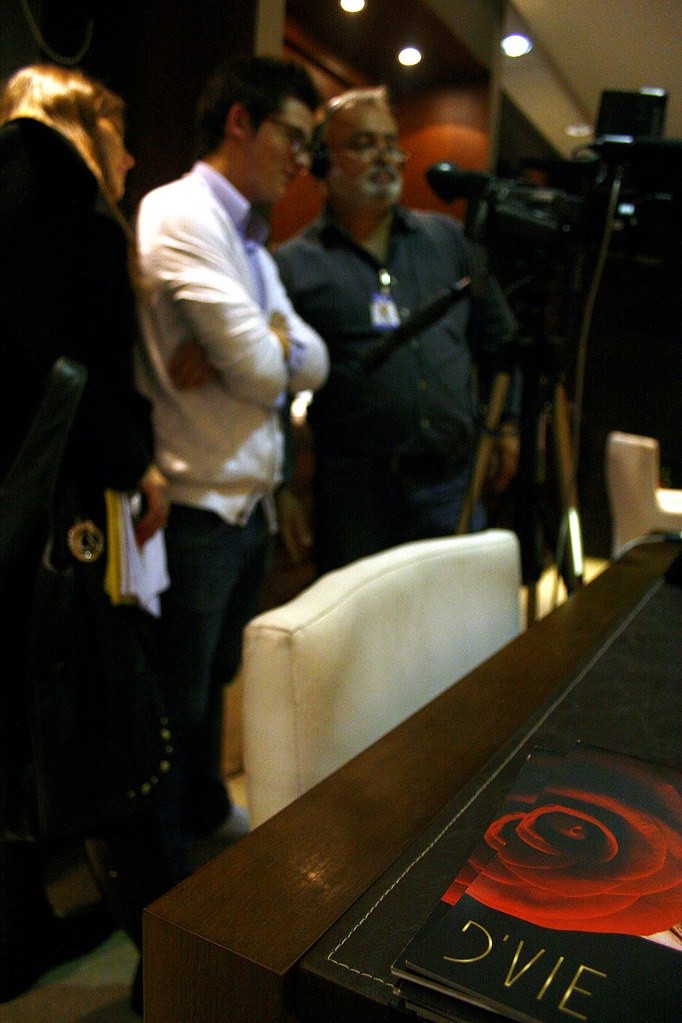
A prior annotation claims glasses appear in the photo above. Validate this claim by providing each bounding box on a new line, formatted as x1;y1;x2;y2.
261;114;314;155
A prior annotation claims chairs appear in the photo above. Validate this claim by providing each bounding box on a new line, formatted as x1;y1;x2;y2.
606;432;682;552
242;528;524;830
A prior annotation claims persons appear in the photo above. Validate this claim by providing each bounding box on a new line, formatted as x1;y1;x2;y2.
122;50;331;963
0;57;178;1009
268;82;526;589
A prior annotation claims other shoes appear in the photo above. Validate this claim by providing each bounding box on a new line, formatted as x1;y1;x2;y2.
203;806;250;839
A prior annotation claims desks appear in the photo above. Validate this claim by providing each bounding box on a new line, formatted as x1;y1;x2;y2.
144;531;682;1023
